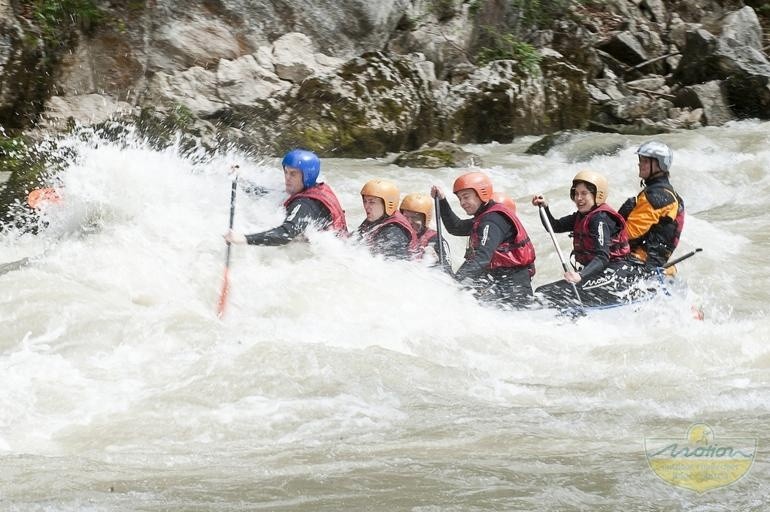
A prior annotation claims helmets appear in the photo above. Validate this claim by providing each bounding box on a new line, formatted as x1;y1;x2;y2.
570;170;609;207
634;140;672;174
492;191;515;214
282;148;320;189
400;194;433;229
361;179;400;216
453;173;494;202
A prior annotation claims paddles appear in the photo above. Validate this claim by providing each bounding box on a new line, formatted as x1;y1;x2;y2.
216;165;239;320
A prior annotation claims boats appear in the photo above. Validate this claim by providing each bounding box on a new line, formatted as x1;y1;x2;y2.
518;274;686;350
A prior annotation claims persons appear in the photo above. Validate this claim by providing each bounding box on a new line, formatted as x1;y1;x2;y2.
344;178;420;264
616;140;686;283
218;148;370;250
531;169;634;311
491;190;517;216
430;170;537;311
399;190;455;281
